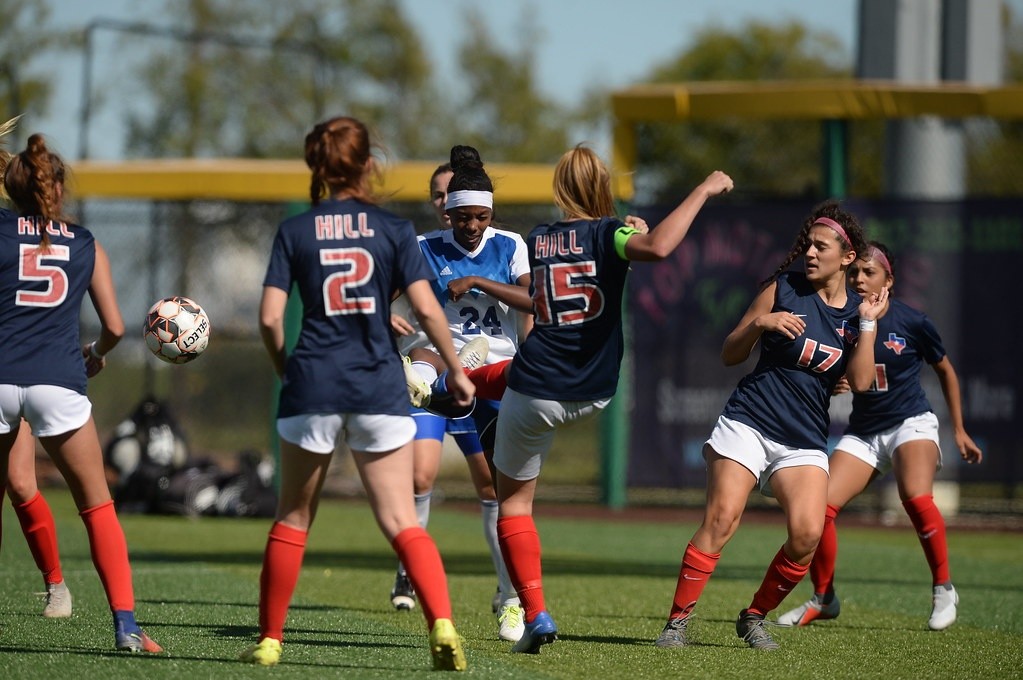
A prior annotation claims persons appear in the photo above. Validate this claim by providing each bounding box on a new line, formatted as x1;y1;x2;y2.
658;203;890;651
238;118;466;671
101;397;277;518
778;241;983;629
433;147;733;653
391;146;527;640
0;133;160;654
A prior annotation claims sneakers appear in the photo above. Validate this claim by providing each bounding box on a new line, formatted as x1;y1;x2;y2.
512;612;557;653
737;609;791;650
492;588;525;641
115;629;163;654
928;583;959;630
43;578;72;618
433;337;489;400
656;600;697;647
429;617;466;672
391;570;415;610
236;637;282;665
777;595;841;626
401;353;430;409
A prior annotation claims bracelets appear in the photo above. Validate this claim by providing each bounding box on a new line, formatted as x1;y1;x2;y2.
859;319;875;331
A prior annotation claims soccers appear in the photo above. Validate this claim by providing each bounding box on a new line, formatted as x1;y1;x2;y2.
142;295;212;365
